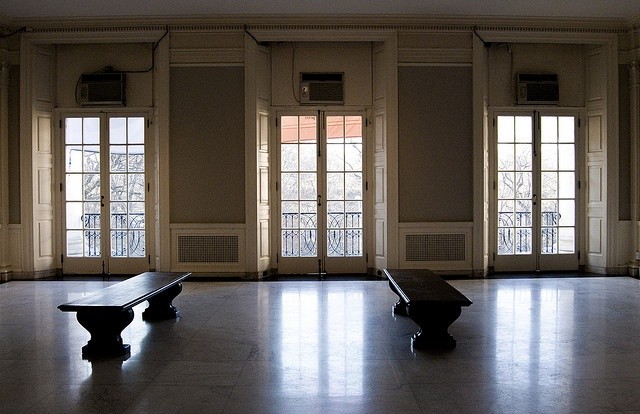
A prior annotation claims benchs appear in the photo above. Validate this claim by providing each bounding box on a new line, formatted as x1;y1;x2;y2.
385;268;474;350
58;272;190;358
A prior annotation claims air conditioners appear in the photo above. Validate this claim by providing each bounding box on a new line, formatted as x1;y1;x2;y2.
514;72;560;105
299;71;346;105
80;72;126;107
